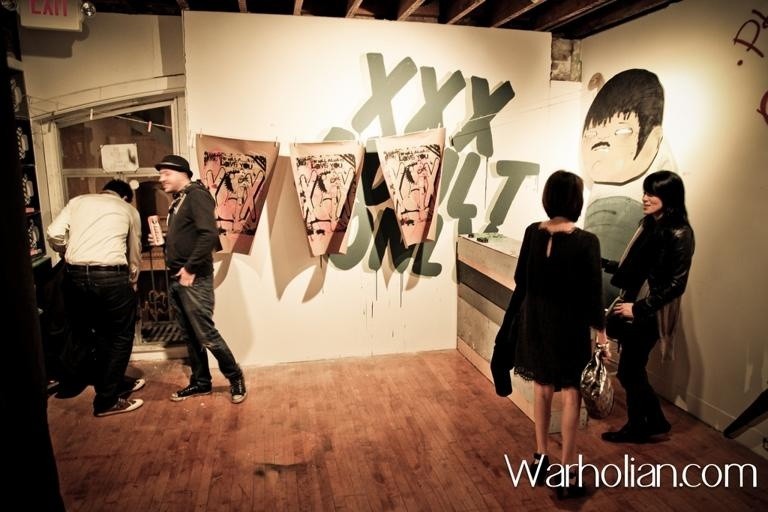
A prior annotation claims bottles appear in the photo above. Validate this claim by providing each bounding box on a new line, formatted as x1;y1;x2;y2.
147;214;165;246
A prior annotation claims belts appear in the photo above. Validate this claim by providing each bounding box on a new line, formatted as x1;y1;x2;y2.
64;262;129;273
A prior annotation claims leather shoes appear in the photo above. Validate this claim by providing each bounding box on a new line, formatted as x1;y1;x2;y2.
601;418;672;444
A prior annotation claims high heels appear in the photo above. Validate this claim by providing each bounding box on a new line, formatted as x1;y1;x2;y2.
554;474;587;501
527;452;550;489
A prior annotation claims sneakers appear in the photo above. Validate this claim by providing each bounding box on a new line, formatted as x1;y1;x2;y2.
117;377;146;396
94;397;144;417
230;373;248;404
169;382;212;402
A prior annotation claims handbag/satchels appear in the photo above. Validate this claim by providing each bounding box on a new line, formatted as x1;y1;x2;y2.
605;296;643;344
578;345;614;421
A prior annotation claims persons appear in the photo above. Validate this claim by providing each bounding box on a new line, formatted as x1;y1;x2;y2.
136;182;172;323
45;180;145;417
148;154;247;403
514;170;612;500
602;170;694;443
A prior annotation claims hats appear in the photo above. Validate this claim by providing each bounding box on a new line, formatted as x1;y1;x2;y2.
155;155;193;177
